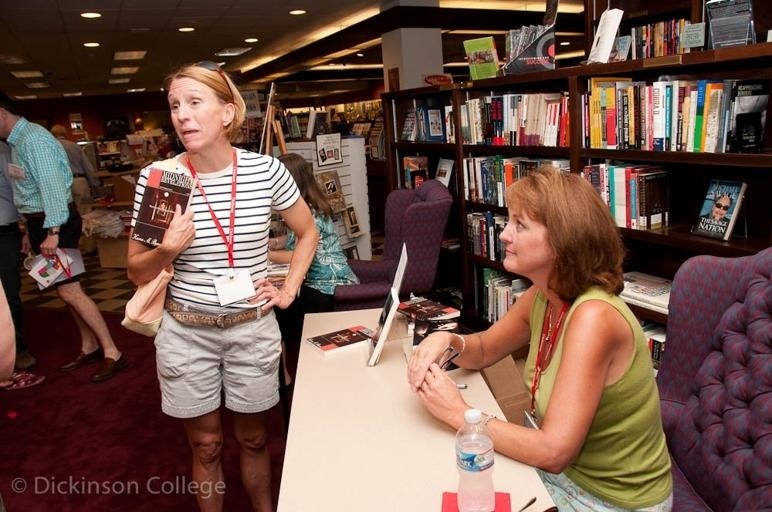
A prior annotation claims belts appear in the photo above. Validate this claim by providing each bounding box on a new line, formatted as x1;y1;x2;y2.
74;173;87;177
165;297;273;329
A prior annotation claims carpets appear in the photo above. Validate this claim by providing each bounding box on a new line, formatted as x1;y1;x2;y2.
0;301;290;512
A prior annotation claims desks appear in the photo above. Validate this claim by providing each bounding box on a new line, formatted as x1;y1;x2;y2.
275;306;555;512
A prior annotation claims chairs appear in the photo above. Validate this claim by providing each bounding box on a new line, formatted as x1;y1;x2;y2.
654;247;772;512
326;178;452;311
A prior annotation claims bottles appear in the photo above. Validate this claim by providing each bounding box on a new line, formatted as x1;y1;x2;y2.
455;408;495;512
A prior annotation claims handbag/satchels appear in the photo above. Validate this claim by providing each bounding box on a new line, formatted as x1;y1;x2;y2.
121;263;175;337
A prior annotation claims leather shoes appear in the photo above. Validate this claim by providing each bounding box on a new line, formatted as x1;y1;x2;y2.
90;351;128;381
62;344;103;370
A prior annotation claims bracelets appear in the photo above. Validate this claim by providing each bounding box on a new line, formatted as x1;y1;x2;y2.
46;229;59;235
453;332;466;361
481;415;495;426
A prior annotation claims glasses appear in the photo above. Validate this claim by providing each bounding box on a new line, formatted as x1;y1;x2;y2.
184;61;234;104
714;202;729;211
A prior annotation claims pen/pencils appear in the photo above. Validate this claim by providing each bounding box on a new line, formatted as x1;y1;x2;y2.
456;384;467;389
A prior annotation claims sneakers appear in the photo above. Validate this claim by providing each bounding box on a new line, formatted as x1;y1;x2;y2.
14;350;36;369
0;370;46;391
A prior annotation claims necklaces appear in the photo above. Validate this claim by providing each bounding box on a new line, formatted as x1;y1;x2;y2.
545;307;557;341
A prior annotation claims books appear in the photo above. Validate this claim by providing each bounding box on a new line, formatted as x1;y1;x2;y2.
397;9;770;383
342;205;363;238
314;170;346;216
341;245;359;260
131;167;198;247
351;100;386;161
80;209;131;239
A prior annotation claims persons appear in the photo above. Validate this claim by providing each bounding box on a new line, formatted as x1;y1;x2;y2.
0;280;17;387
1;141;37;371
0;86;128;382
51;125;104;256
402;166;674;512
126;61;320;512
263;152;360;389
